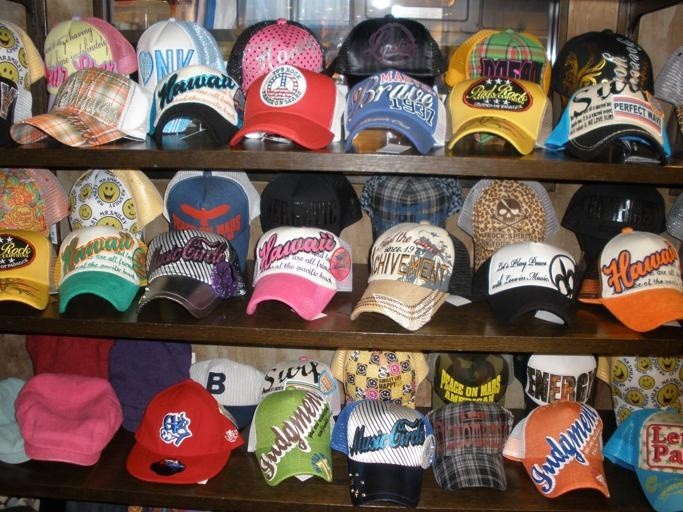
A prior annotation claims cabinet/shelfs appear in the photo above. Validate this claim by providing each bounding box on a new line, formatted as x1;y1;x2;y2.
0;1;682;508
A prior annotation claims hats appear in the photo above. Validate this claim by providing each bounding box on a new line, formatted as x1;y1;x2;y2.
331;348;430;410
501;399;612;499
148;64;247;146
328;397;436;510
349;219;473;332
0;19;46;127
228;63;346;149
359;172;463;238
549;29;655;101
189;358;266;433
544;79;673;165
472;240;584;329
67;169;169;247
576;226;683;333
137;228;247;320
443;75;554;156
425;400;515;492
326;14;449;87
1;376;31;465
600;407;683;512
343;68;447;155
125;378;244;486
0;168;70;233
560;181;667;262
245;226;353;322
48;224;149;314
163;168;261;274
108;336;192;433
512;351;599;417
44;16;138;95
9;67;148;148
226;17;327;98
444;28;552;97
25;333;115;381
246;385;334;486
14;371;123;467
456;177;561;273
596;354;683;427
0;229;59;311
260;355;342;417
431;351;511;410
136;16;229;93
260;170;363;237
